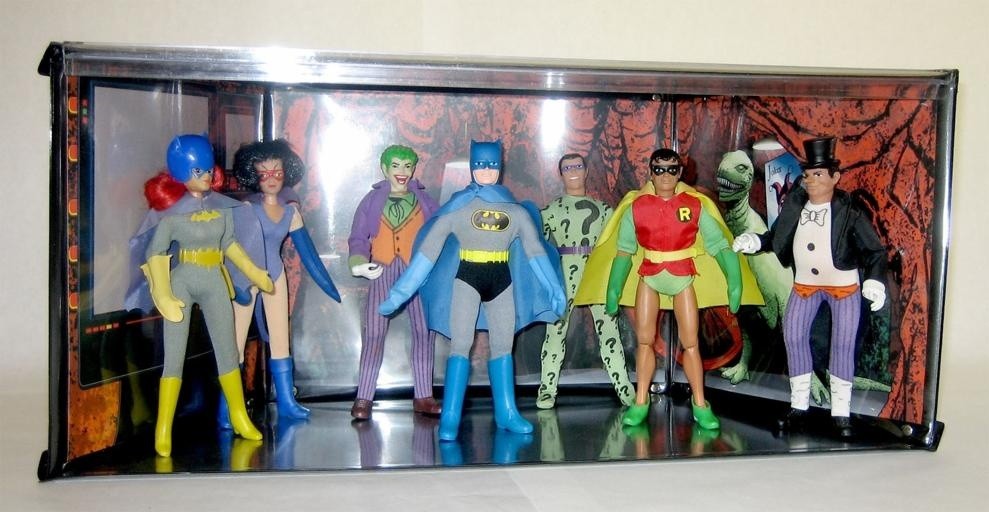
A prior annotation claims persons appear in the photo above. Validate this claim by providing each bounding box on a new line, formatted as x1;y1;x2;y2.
347;147;446;423
139;133;275;458
605;148;743;432
218;137;341;432
378;139;570;443
733;132;888;436
537;155;637;407
